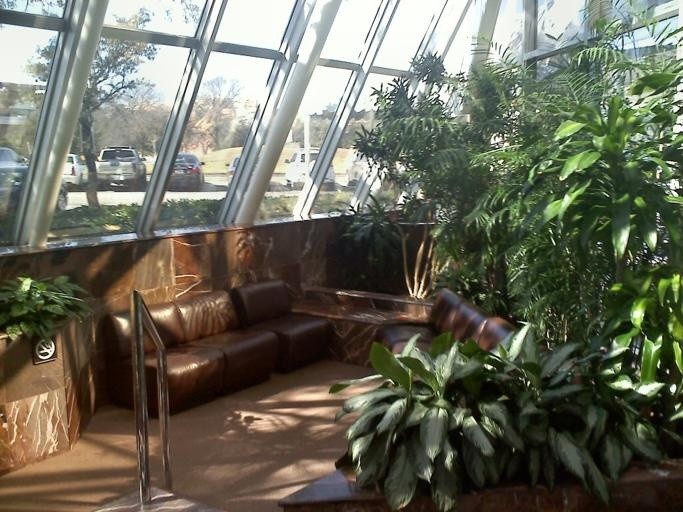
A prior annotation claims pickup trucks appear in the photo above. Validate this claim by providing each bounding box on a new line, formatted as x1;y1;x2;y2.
93;146;145;190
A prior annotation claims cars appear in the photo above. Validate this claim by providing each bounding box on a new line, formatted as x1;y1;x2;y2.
0;147;27;164
164;152;205;191
345;149;405;189
224;153;240;185
0;160;68;213
61;153;82;190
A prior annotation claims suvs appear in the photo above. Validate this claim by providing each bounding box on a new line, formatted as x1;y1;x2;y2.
282;146;334;190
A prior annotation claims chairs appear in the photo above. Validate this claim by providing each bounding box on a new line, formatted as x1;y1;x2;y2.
106;301;225;418
172;291;273;395
231;278;331;371
